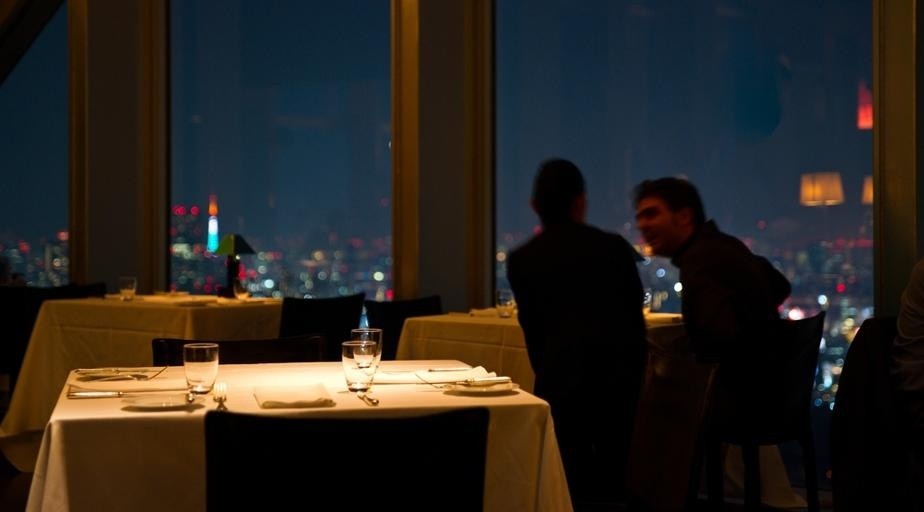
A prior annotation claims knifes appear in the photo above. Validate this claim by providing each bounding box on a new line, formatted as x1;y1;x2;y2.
355;390;379;405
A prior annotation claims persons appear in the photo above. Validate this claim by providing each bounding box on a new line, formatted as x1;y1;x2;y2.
631;178;792;512
505;156;649;512
829;254;924;512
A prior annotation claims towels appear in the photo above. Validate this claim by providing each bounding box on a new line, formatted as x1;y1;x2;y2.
253;382;336;409
417;366;519;394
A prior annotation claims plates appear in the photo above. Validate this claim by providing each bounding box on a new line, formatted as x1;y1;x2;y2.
445;382;520;396
120;395;205;409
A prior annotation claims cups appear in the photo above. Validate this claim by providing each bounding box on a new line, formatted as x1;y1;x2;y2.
119;277;137;301
351;328;382;367
342;341;376;392
183;342;219;394
233;278;249;299
495;289;513;318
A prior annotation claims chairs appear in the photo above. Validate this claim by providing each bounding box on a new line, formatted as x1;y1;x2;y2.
0;281;107;400
279;292;366;361
834;317;897;512
705;310;828;512
151;336;323;366
203;409;491;512
364;295;443;349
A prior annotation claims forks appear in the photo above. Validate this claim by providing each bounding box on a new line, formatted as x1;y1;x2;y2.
212;395;227;413
